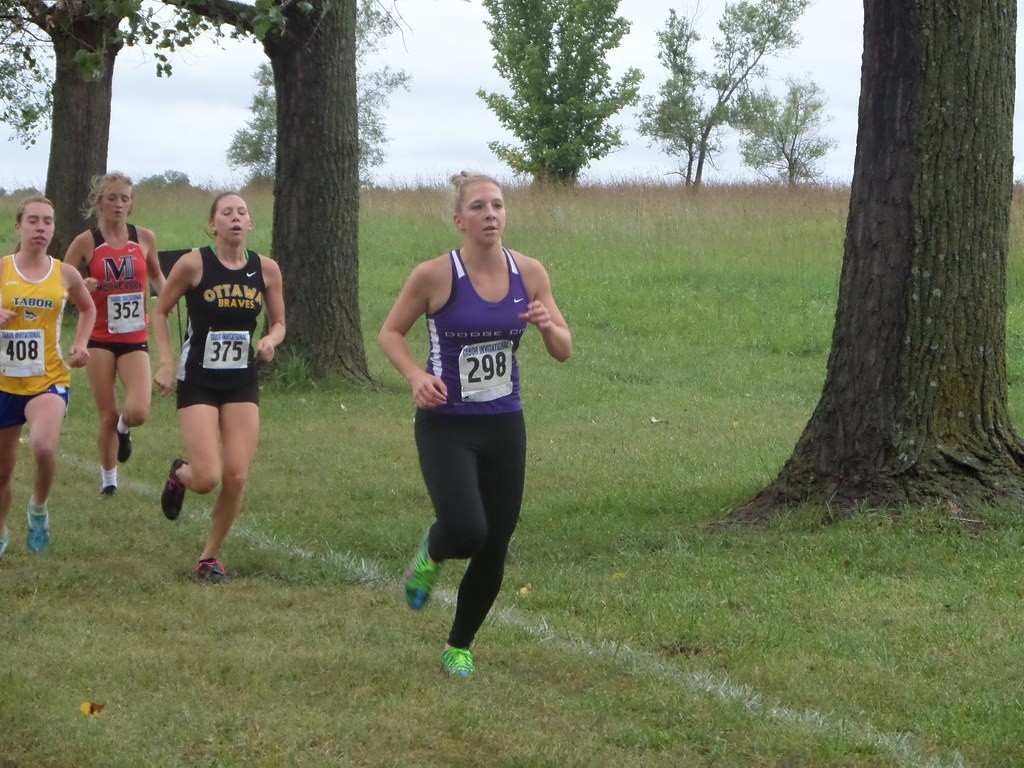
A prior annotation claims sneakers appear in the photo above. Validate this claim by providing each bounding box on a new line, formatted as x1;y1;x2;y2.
100;484;118;498
440;646;478;681
116;422;133;463
0;527;8;556
191;557;230;585
160;457;194;521
26;495;50;554
403;520;448;611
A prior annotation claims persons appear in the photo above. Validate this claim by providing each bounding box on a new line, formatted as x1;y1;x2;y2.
378;171;572;678
151;191;285;584
0;195;96;558
64;171;175;500
